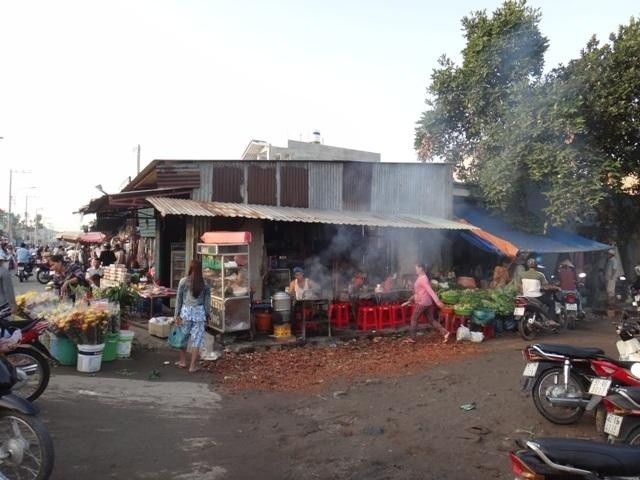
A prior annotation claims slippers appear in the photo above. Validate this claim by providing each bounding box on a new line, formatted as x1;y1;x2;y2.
442;332;452;344
403;338;418;344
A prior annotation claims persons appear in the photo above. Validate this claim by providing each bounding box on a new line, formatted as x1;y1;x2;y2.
401;262;450;345
171;257;213;375
287;266;322;304
489;248;619;328
1;227;132;293
231;255;256;310
1;254;18;329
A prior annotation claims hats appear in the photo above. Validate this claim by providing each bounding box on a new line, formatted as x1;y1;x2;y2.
560;259;576;269
608;249;616;256
293;266;304;273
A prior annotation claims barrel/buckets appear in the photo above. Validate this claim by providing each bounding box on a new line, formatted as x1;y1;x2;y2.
51;329;135;373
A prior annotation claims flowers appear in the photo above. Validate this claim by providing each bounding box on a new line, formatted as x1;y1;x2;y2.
14;281;140;346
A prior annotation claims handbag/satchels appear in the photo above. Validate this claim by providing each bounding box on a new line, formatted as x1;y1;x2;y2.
168;326;190;350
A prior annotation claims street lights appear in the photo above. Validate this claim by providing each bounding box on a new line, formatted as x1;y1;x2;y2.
24;185;38;248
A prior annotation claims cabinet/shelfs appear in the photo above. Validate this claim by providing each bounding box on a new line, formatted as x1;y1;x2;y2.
196;242;253;346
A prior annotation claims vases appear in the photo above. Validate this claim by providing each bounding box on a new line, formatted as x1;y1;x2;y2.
49;330;135;373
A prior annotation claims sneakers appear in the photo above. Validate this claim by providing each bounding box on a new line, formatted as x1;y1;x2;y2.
174;360;200;372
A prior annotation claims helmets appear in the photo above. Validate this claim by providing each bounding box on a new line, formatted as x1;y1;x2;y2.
527;258;536;268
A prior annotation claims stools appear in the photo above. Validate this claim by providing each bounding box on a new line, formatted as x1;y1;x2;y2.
296;302;467;333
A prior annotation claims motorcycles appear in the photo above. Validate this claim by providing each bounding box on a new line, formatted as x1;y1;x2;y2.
510;272;640;480
0;306;54;480
15;254;52;284
